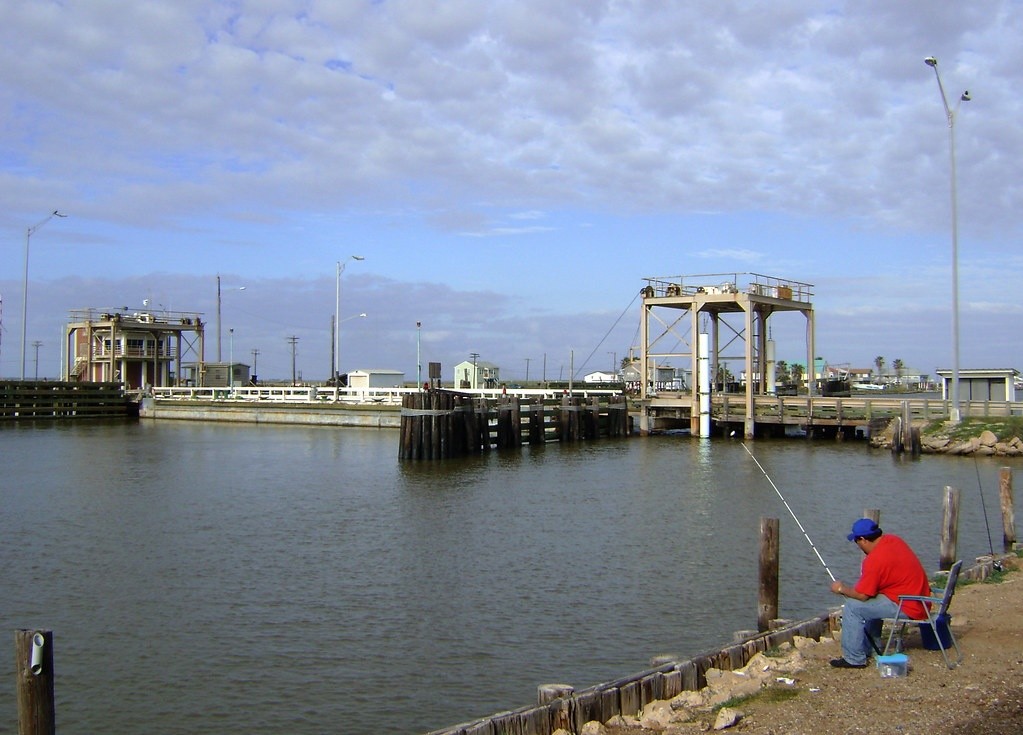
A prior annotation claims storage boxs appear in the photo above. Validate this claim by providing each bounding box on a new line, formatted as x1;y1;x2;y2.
877;653;909;678
918;614;951;651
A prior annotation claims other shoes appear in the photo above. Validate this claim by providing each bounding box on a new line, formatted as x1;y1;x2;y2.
830;657;866;668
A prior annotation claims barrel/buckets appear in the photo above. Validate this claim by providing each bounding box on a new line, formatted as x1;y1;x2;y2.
920;614;953;652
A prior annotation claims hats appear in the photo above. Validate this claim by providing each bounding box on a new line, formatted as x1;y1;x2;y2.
847;518;881;542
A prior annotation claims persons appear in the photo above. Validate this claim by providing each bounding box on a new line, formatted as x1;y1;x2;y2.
828;519;931;669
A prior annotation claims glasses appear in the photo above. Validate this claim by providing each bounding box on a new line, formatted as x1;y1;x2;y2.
854;538;861;544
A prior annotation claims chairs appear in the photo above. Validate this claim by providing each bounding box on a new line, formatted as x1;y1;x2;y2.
882;559;963;669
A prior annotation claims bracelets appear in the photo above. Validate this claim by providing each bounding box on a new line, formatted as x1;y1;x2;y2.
838;585;843;593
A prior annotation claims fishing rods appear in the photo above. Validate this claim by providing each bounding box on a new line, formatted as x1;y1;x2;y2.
738;438;885;657
969;436;1005;573
